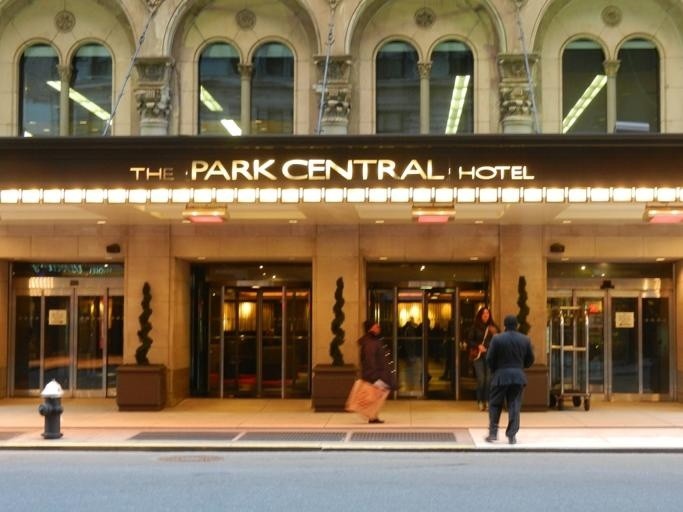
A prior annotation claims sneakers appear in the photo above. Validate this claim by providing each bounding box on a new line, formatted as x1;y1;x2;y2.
487;434;497;441
509;435;516;444
479;399;487;411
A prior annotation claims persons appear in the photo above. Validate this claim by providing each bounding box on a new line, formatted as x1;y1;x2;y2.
465;307;500;410
355;319;391;424
381;317;447;365
484;315;535;445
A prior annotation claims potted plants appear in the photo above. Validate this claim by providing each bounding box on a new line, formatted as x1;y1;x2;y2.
504;276;549;413
312;277;360;412
116;282;168;411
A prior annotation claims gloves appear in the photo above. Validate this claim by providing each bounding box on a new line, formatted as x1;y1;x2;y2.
375;379;387;389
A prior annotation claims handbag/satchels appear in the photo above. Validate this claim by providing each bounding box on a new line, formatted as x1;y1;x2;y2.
470;346;481;361
344;378;390;421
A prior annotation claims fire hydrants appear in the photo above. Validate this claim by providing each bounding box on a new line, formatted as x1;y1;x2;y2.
39;379;67;440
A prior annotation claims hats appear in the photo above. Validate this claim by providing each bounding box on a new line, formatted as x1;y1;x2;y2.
363;320;376;330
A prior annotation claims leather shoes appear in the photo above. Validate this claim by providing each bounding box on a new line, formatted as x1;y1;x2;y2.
369;418;384;424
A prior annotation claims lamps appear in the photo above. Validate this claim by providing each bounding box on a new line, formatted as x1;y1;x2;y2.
180;203;232;224
219;119;242;137
410;205;456;224
199;84;224;113
444;75;471;134
563;74;607;135
45;80;113;128
644;201;683;224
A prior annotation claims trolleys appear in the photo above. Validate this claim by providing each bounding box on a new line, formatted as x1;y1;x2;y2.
546;306;590;412
